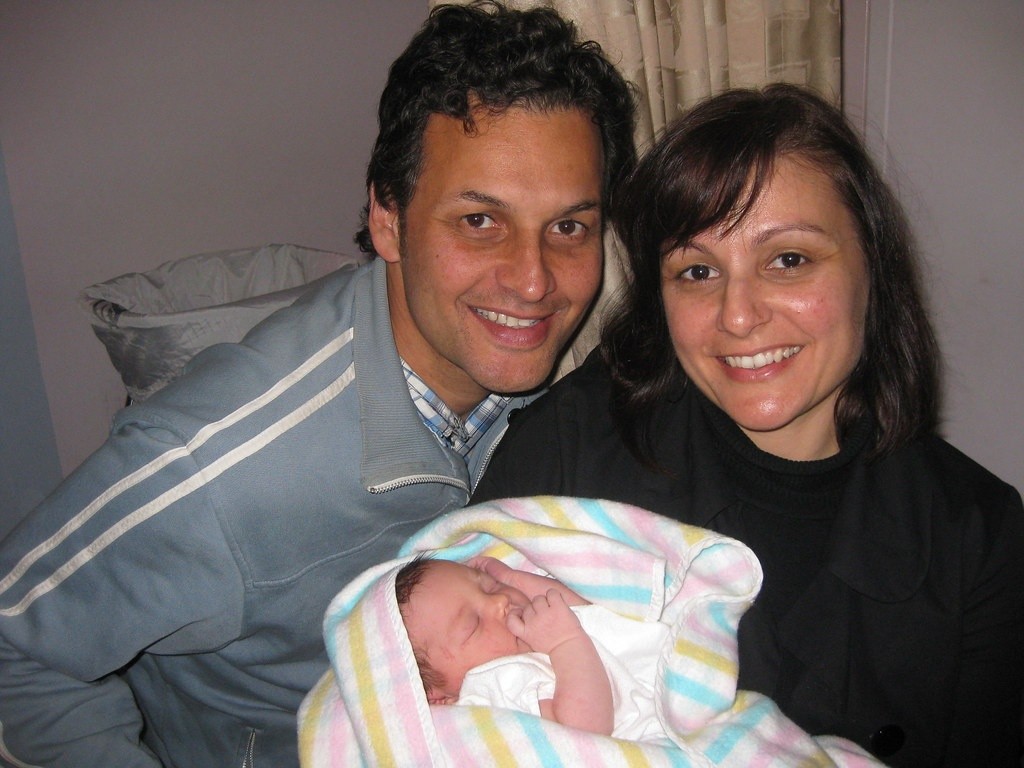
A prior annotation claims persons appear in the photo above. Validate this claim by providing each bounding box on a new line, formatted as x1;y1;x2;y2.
462;83;1024;768
394;549;673;741
0;0;638;768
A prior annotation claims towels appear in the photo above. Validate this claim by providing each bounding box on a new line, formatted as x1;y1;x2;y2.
295;496;894;768
